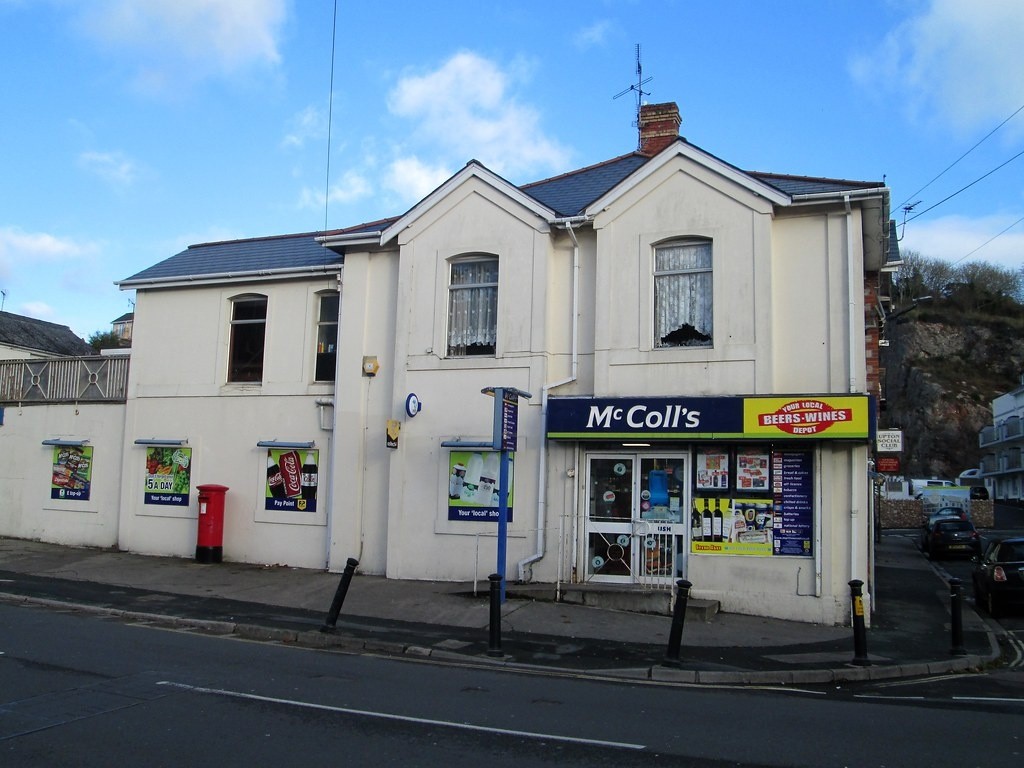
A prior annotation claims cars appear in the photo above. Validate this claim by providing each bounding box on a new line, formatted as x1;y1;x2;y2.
874;464;989;501
927;519;981;561
971;537;1024;615
927;506;968;529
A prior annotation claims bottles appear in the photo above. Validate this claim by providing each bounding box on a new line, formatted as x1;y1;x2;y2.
53;464;90;492
700;498;712;541
690;498;702;541
459;449;484;503
713;469;718;487
450;462;467;499
266;450;286;499
475;450;500;507
721;467;726;487
712;498;723;542
735;501;767;534
300;449;317;500
648;459;669;509
489;456;514;507
